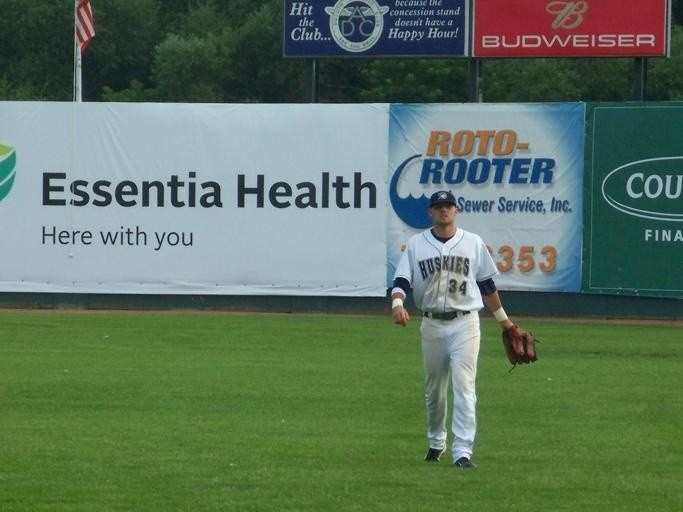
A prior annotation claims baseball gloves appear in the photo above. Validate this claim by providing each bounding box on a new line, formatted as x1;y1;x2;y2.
502;324;537;365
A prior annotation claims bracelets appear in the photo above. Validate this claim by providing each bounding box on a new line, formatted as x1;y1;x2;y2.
492;306;508;323
391;298;404;310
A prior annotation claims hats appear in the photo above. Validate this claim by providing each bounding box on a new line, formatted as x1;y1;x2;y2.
431;191;456;205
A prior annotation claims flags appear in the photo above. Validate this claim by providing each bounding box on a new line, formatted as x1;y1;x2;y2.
74;0;96;56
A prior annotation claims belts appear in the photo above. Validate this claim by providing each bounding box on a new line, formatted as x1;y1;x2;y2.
424;312;470;320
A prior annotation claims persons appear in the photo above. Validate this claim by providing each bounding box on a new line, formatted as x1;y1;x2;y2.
390;190;541;467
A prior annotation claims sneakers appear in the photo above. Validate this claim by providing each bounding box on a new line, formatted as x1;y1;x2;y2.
424;443;446;462
454;457;478;468
